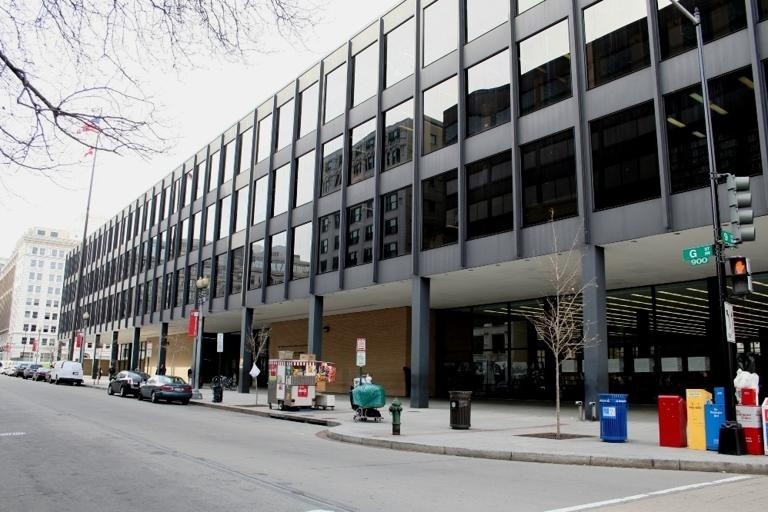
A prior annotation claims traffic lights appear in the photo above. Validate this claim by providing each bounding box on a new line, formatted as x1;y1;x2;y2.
727;175;755;244
729;255;753;296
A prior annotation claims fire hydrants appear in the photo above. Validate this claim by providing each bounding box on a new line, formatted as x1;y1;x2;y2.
389;398;402;435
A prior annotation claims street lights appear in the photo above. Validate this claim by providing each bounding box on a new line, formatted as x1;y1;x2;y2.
81;312;89;367
192;275;209;399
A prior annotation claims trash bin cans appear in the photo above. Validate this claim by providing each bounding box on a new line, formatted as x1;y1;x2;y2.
658;396;685;449
598;393;628;443
213;385;222;402
449;391;472;430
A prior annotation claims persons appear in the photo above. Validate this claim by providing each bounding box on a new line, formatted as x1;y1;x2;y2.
158;363;164;375
98;367;102;381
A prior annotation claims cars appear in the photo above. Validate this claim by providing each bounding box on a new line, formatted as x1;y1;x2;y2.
7;360;83;385
108;370;150;396
138;375;192;404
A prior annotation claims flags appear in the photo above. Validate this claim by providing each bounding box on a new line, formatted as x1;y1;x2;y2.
31;339;38;352
188;309;199;337
75;332;84;348
